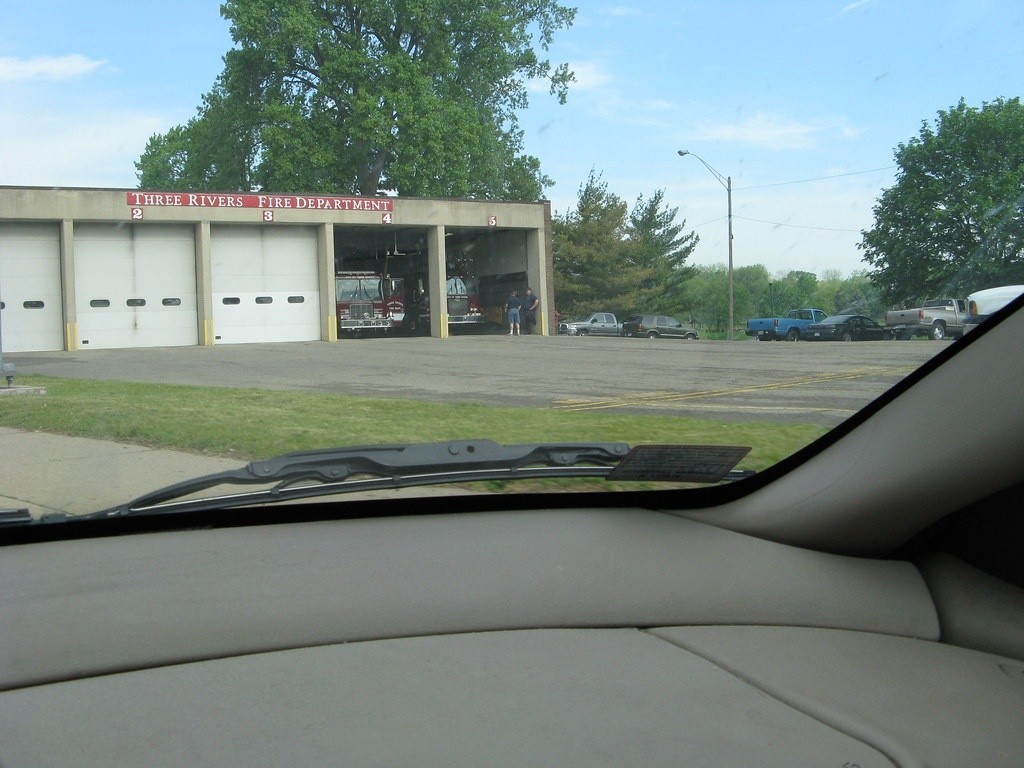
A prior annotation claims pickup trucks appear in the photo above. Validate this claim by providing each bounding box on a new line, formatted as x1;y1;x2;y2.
745;308;830;342
882;297;969;342
569;312;623;337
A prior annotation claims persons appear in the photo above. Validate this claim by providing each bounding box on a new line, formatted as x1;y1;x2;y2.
505;289;539;335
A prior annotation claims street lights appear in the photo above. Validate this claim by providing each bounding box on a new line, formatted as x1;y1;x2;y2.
678;149;734;340
769;282;774;318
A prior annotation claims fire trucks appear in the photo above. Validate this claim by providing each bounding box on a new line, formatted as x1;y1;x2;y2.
334;267;413;339
405;255;488;332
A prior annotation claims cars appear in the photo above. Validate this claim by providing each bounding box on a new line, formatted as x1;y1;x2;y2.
806;314;895;341
963;284;1024;338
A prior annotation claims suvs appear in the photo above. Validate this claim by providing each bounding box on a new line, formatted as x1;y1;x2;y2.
626;313;700;341
